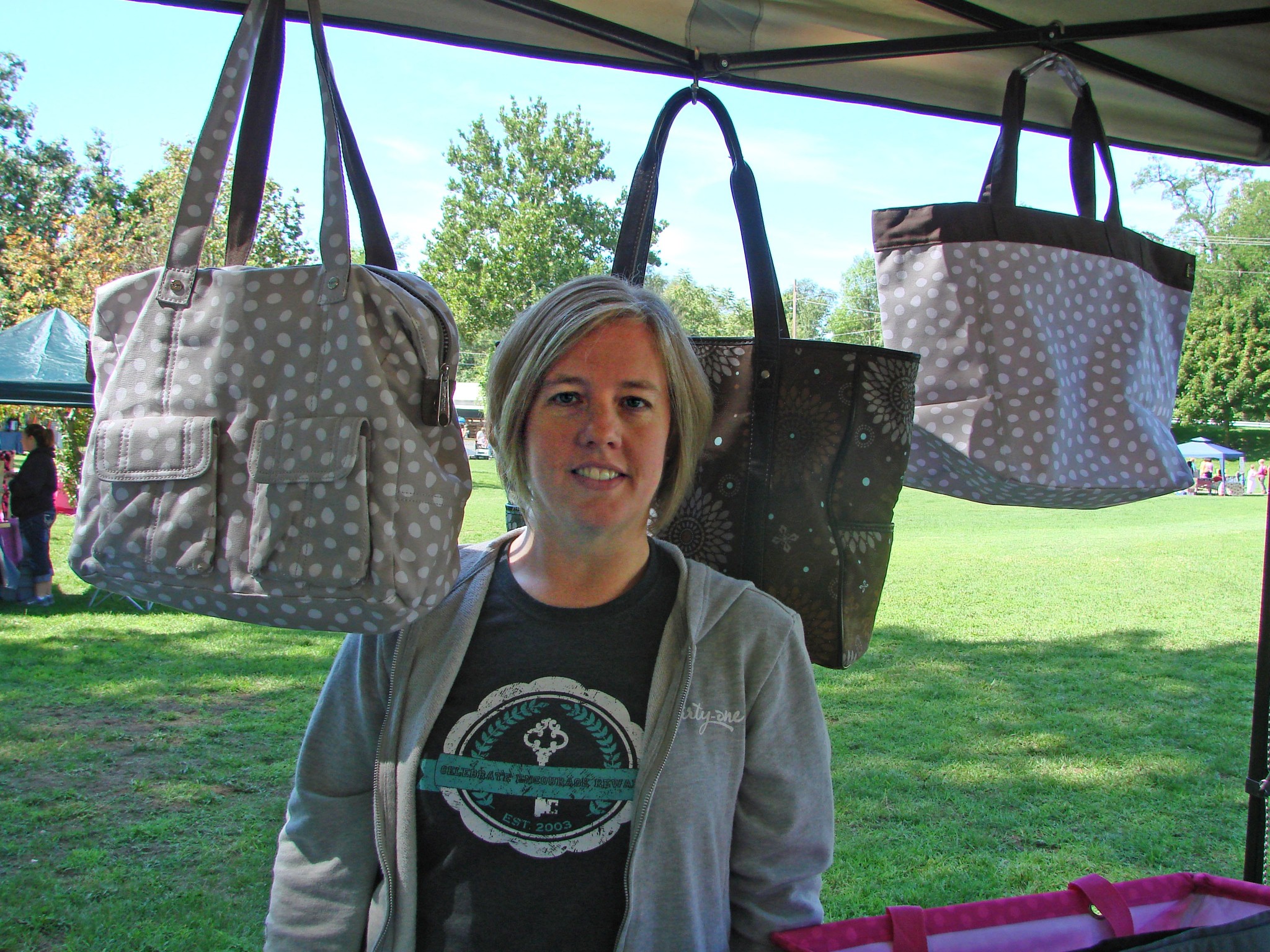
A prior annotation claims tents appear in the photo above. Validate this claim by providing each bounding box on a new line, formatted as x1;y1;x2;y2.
1177;436;1245;496
0;307;95;406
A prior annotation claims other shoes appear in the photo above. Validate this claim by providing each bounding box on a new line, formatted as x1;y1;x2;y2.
20;592;55;607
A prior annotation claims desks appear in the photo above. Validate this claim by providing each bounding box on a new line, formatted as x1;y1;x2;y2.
463;438;477;456
1212;481;1243;496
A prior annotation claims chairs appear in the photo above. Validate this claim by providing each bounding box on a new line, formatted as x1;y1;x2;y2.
1197;477;1212;494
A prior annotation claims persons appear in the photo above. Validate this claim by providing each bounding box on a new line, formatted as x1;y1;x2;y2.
1186;458;1270;497
261;275;837;952
9;424;57;607
457;423;497;458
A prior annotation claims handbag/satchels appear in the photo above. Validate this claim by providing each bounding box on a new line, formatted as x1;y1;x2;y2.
771;871;1270;952
66;0;470;635
505;87;921;669
869;53;1196;510
